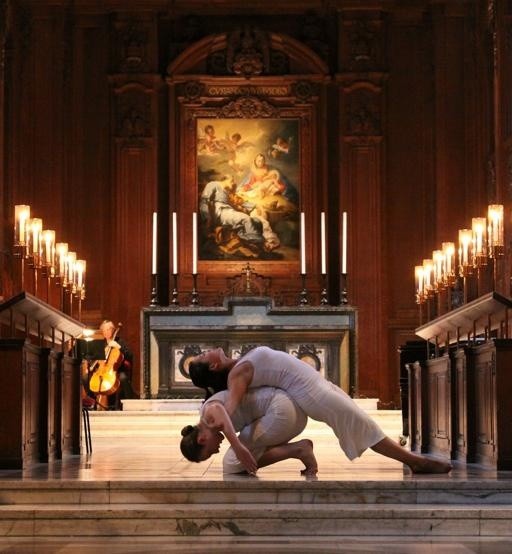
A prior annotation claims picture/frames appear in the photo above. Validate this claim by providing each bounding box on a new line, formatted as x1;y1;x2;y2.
179;104;316;279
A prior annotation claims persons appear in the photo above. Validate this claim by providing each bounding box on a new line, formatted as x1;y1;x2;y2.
80;316;132;397
187;344;453;473
198;172;259;242
207;132;242;150
242;151;290;198
180;386;319;475
196;120;217;153
249;203;279;251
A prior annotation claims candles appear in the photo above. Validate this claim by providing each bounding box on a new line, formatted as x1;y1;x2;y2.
152;212;158;274
192;212;198;274
342;212;347;274
300;212;306;274
321;212;326;274
172;212;178;274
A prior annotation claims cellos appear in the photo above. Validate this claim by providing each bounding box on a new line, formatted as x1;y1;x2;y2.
88;322;125;394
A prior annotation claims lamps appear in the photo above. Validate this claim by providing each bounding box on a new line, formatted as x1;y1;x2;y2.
413;205;512;359
0;204;87;358
